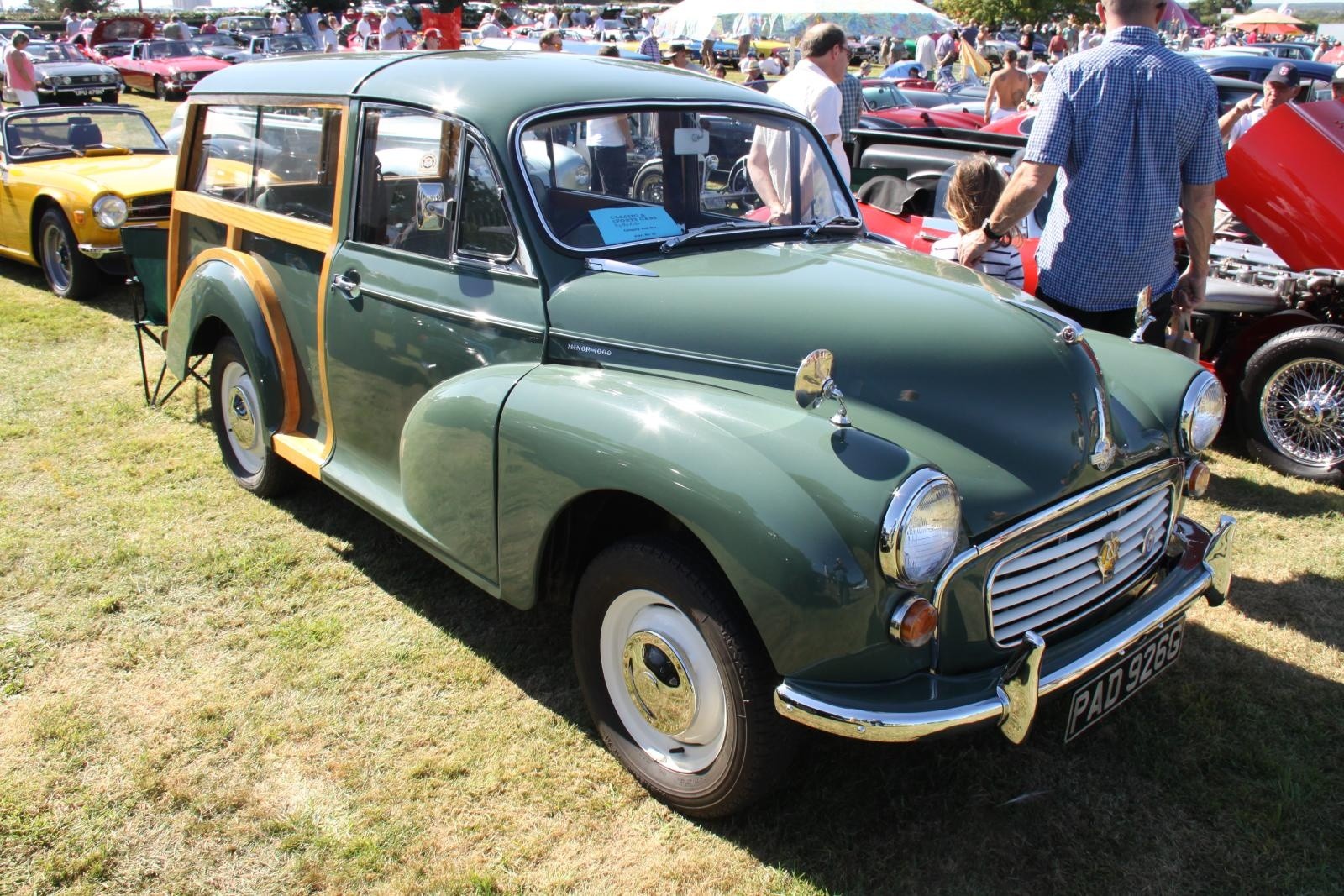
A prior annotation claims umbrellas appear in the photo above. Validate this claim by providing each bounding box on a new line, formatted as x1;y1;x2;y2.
1227;8;1304;33
960;37;992;79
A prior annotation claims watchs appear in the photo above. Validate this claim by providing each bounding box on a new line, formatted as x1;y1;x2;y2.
983;217;1004;240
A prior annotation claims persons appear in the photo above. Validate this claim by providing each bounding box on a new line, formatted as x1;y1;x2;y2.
142;12;217;40
4;33;44;133
586;45;634;199
859;18;1106;124
534;33;569;145
1158;28;1344;99
747;23;852;223
1219;63;1302;150
269;6;448;53
957;0;1229;348
33;8;97;38
931;158;1024;290
476;5;784;94
834;73;863;167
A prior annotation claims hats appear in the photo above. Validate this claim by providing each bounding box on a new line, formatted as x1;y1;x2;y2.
387;6;398;15
663;43;693;58
641;9;649;14
1025;61;1048;74
87;10;95;16
1319;34;1330;42
590;11;598;17
860;62;873;71
1324;63;1344;88
70;13;77;16
1263;63;1301;88
934;30;941;36
205;16;215;21
425;28;448;42
748;60;760;71
747;50;757;57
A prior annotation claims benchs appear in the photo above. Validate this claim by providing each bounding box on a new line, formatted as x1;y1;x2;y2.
255;177;451;219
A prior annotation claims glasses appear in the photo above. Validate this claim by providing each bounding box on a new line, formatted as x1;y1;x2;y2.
839;46;854;60
551;43;562;50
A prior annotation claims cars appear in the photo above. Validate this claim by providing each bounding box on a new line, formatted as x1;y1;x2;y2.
119;49;1234;822
612;41;1344;483
0;13;591;299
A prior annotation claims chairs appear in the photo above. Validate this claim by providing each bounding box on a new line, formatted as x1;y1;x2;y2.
68;123;104;153
118;224;215;406
6;125;24;157
47;51;59;60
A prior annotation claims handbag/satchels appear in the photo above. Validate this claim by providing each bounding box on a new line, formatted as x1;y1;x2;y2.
1020;35;1029;47
1165;311;1201;363
